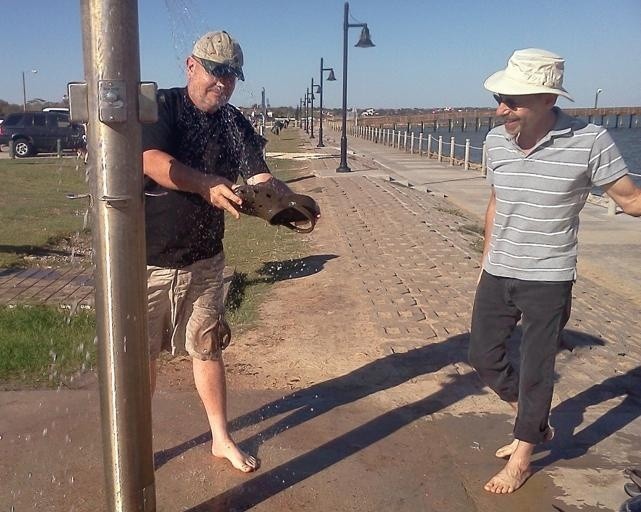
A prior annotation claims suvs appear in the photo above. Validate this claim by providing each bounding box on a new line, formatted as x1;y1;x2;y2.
0;113;87;158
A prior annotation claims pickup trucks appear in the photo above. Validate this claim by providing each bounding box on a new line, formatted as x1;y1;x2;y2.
444;107;453;112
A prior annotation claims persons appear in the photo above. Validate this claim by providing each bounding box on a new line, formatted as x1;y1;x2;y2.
144;29;321;473
468;48;641;494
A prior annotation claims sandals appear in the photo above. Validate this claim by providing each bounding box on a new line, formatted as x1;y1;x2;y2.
624;468;641;497
230;178;320;233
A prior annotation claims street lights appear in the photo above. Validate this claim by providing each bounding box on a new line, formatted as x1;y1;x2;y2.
595;89;602;109
22;69;39;112
300;58;336;148
335;2;375;172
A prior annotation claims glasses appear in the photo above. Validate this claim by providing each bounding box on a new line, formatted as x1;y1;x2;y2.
493;94;529;111
192;56;231;78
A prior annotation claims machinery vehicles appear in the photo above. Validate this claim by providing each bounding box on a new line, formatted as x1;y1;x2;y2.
360;109;375;117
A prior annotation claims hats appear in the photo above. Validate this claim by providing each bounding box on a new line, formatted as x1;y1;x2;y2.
484;49;575;102
192;31;245;81
